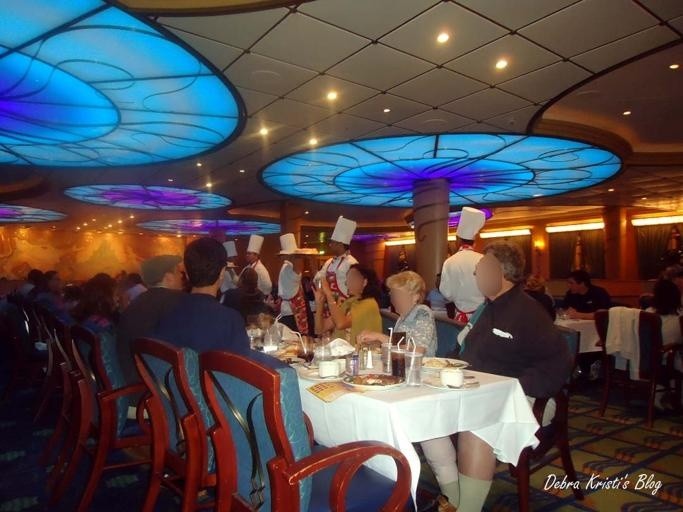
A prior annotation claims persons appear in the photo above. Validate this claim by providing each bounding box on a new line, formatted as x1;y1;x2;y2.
643;280;683;409
19;207;576;512
639;294;657;311
557;269;615;383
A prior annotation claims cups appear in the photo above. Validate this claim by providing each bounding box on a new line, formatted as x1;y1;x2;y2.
380;343;398;374
390;349;405;378
405;351;423;387
245;326;330;368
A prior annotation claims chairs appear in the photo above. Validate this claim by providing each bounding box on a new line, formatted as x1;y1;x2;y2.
1;289;683;512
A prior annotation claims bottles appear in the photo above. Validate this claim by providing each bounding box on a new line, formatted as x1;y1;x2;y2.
344;339;380;374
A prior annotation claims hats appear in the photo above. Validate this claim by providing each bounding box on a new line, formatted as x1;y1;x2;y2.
141;255;181;283
247;235;264;255
280;233;297;249
331;216;357;245
457;207;486;240
223;241;237;257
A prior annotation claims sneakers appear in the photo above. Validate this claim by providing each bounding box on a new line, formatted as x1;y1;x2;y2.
422;494;456;512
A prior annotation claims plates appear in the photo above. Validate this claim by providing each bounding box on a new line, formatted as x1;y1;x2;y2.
420;356;470;371
342;374;407;391
296;370;346;384
419;369;481;391
